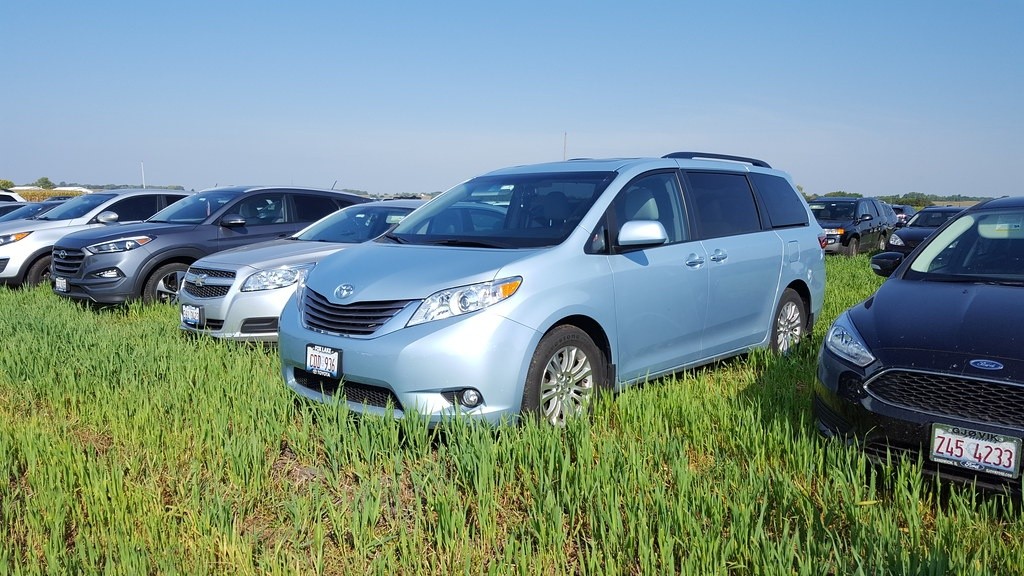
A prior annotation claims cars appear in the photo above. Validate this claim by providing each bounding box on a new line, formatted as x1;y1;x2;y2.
0;201;66;221
44;196;72;201
812;196;1024;497
178;200;509;343
886;207;972;260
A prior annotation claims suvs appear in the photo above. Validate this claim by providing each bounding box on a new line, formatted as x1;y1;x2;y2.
0;189;27;202
806;198;888;258
277;152;827;440
892;205;918;228
0;188;197;291
880;201;897;230
51;186;378;309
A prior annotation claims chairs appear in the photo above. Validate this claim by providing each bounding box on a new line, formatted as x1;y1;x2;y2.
818;210;830;219
624;189;669;245
272;202;284;223
240;202;262;225
932;212;942;218
849;206;855;218
697;195;730;239
544;191;568;220
